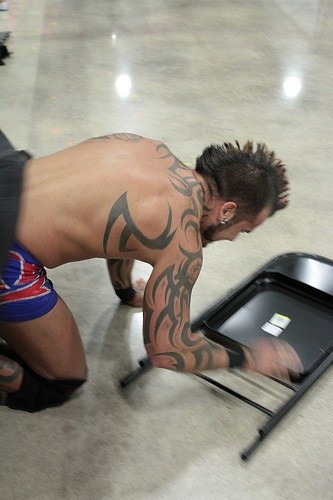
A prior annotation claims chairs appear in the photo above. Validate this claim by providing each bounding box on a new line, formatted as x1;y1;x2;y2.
119;251;333;458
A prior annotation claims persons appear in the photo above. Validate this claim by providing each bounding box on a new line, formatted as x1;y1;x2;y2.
1;131;308;412
